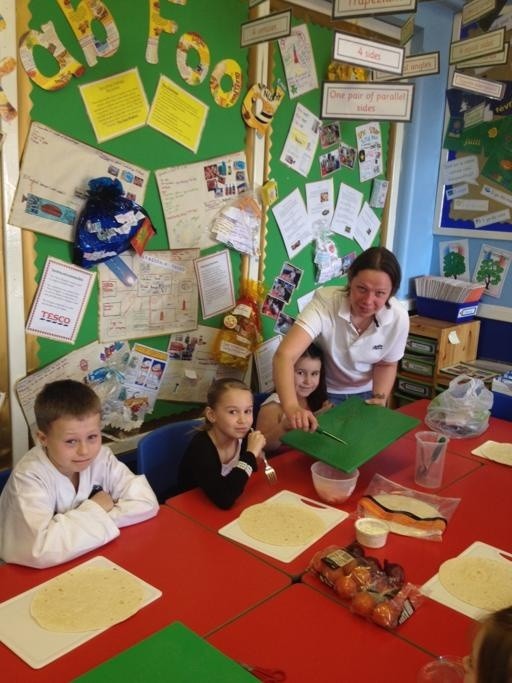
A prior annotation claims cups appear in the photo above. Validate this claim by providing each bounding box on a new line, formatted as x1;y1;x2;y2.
414;654;467;683
414;430;449;489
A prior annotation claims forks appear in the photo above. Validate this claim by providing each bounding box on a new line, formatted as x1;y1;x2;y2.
249;426;279;486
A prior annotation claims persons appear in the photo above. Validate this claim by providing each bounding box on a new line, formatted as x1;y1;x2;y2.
462;605;512;682
1;379;160;570
273;248;411;435
256;343;332;453
172;378;266;509
319;125;356;175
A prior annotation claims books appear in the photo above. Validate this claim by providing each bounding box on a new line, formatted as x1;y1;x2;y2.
415;275;487;303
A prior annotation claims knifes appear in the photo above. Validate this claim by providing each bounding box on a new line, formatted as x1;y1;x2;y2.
309;423;348;446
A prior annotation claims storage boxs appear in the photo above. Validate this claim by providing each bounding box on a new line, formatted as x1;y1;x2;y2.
415;294;482;322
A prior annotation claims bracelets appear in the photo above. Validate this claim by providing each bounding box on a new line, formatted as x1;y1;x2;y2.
372;394;384;398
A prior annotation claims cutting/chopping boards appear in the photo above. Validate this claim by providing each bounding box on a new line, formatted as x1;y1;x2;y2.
218;488;350;565
470;437;512;468
279;394;421;475
0;555;164;670
416;539;511;623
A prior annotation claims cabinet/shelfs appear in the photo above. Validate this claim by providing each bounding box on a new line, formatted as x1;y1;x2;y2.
386;314;482;410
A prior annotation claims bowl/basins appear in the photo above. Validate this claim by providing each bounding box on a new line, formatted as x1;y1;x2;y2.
354;516;389;550
309;460;360;504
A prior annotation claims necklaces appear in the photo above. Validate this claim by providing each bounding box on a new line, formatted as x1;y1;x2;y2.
351;319;368;334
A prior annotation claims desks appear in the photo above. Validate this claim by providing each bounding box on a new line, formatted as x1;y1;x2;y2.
1;399;512;683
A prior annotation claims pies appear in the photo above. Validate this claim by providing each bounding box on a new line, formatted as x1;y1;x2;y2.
240;503;325;547
365;494;443;537
29;568;143;633
481;444;512;466
440;557;512;612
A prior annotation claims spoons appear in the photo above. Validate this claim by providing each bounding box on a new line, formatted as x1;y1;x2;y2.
417;436;444;483
